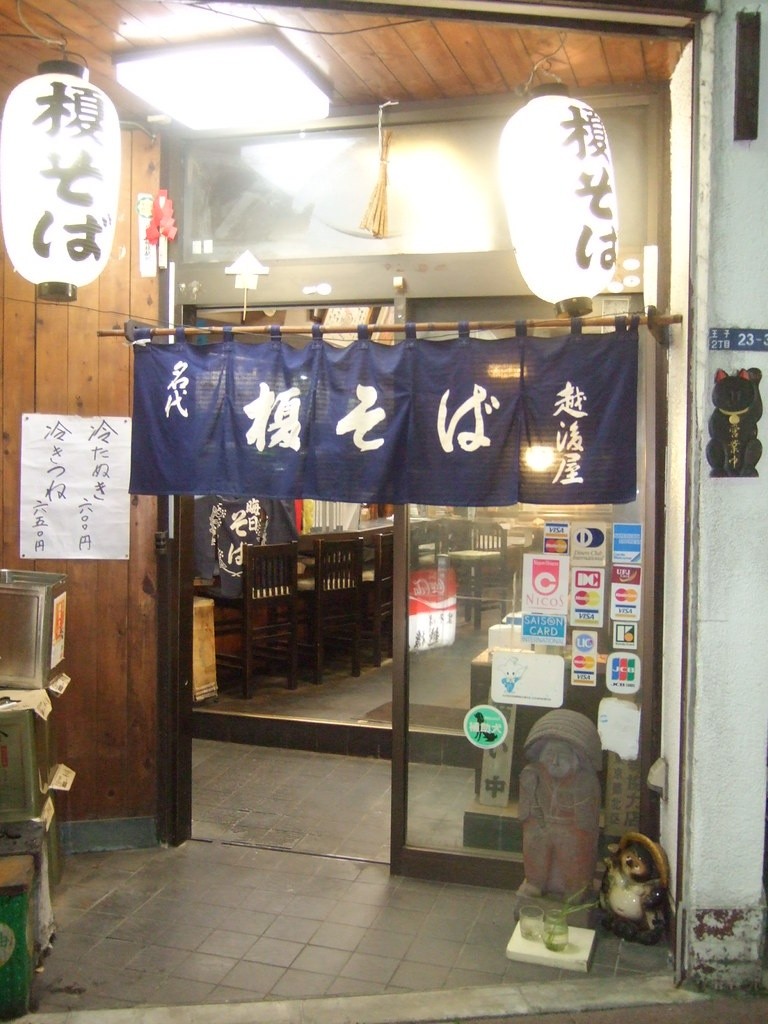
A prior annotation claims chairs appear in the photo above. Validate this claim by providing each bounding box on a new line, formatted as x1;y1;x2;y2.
197;521;508;698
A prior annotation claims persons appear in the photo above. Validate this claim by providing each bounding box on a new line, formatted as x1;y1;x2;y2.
518;708;604;902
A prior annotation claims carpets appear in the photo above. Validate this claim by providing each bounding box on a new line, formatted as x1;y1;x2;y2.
363;699;469;730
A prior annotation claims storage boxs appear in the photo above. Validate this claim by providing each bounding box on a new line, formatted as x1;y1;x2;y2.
0;567;76;1018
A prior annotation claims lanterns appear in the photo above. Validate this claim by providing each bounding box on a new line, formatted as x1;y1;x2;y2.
0;60;121;301
498;82;617;318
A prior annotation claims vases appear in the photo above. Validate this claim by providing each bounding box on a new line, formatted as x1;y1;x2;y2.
518;905;544;941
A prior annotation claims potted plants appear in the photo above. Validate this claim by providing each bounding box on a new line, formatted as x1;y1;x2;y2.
541;884;600;952
